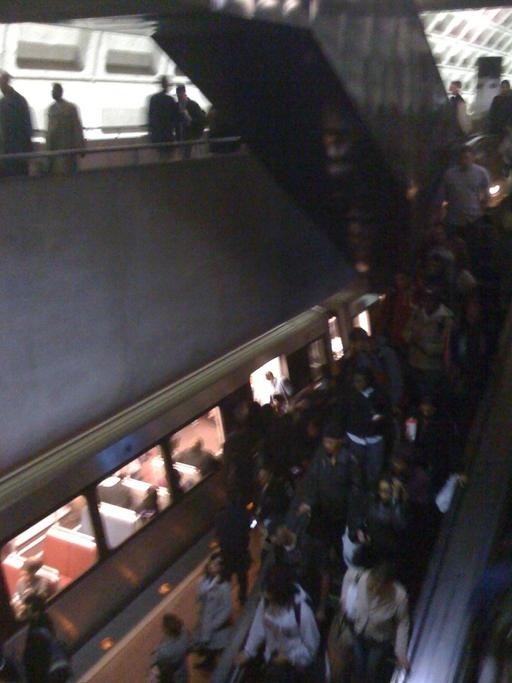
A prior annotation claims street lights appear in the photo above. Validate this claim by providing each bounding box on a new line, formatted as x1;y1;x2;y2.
325;609;358;682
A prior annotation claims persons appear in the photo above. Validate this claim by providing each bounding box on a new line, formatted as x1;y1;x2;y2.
171;84;206;160
0;69;35;176
146;608;192;682
12;560;49;614
422;77;512;287
137;486;159;521
21;594;59;679
148;74;190;164
42;82;86;177
203;103;247;160
172;243;488;681
